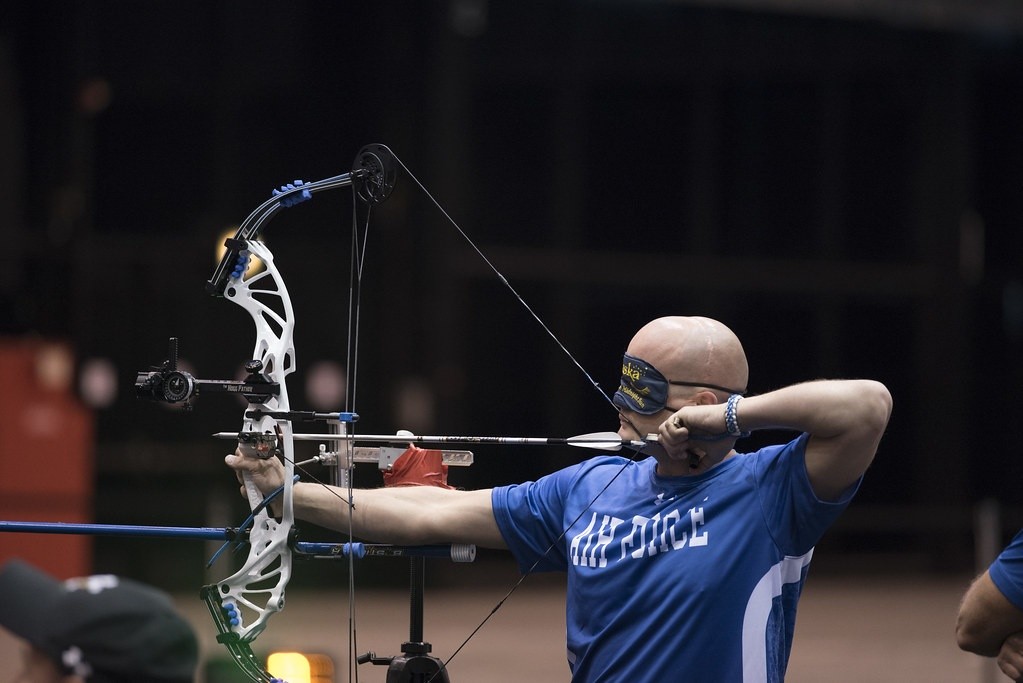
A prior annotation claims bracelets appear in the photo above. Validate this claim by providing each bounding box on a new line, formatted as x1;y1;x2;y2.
725;394;751;437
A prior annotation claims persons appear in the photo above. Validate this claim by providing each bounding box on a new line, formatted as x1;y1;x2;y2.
0;560;200;683
224;315;893;683
954;528;1023;681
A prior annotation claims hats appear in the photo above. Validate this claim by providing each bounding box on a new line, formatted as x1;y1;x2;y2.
0;559;198;683
